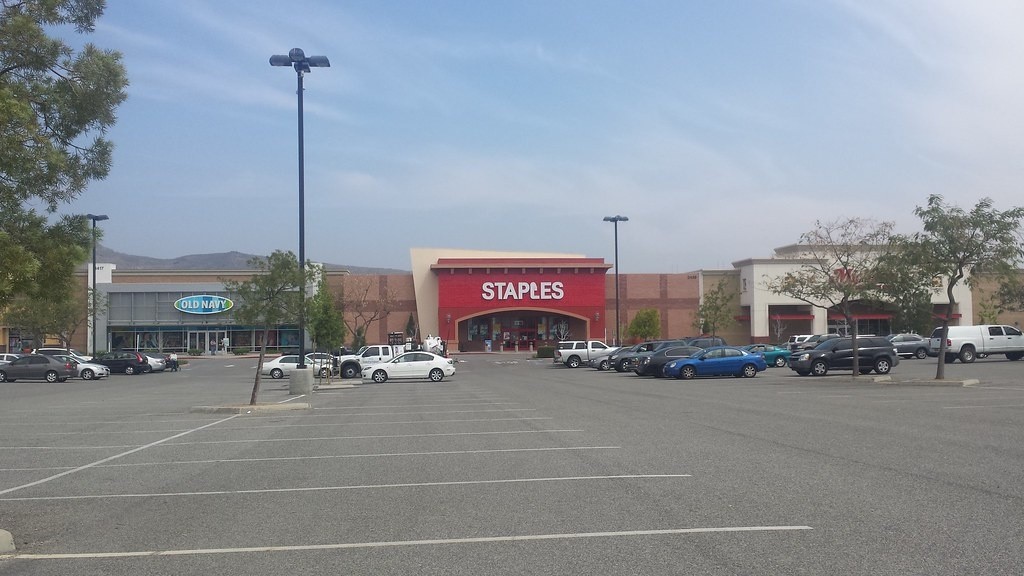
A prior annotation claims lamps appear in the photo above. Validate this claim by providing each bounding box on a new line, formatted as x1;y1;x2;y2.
595;311;600;321
446;315;451;323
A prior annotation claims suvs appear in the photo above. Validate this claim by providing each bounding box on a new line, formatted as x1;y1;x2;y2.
787;336;902;378
778;333;841;356
607;339;688;373
30;348;97;362
685;335;728;353
85;351;148;375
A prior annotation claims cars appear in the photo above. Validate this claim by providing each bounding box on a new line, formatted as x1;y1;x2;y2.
884;333;930;360
590;346;646;371
124;352;166;374
0;353;24;365
148;353;180;369
738;343;792;369
0;354;79;383
663;346;767;380
51;355;111;380
304;352;342;378
360;351;456;383
635;346;715;378
262;355;334;379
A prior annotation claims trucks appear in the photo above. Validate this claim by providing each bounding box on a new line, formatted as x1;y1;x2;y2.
334;345;412;379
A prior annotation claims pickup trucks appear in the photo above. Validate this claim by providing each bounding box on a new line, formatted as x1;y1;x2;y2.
554;340;622;369
928;324;1023;363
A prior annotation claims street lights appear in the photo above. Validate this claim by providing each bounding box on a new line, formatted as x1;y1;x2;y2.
603;215;629;350
86;212;109;359
269;47;331;365
